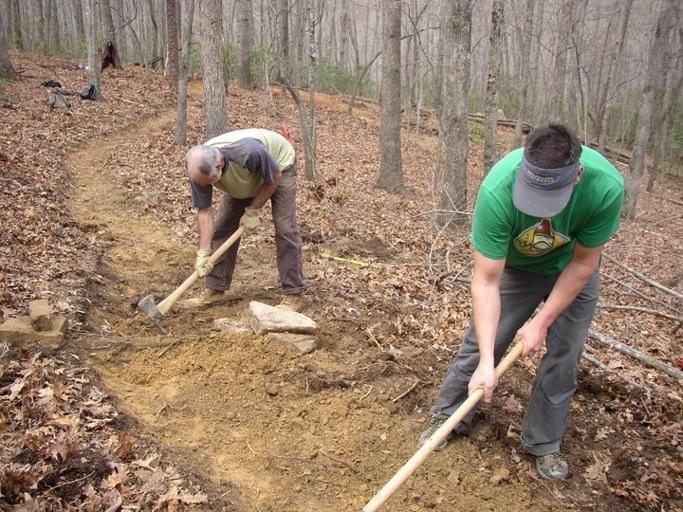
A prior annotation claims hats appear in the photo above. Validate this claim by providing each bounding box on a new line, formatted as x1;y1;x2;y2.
511;150;581;219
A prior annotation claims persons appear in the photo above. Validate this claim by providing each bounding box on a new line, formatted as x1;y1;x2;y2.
184;127;306;314
416;121;625;482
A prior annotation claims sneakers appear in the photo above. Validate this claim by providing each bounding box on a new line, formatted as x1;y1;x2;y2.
182;287;225;309
417;413;469;453
535;449;570;482
274;292;308;313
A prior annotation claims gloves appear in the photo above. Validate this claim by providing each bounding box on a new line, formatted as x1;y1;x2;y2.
193;247;214;278
239;207;262;238
